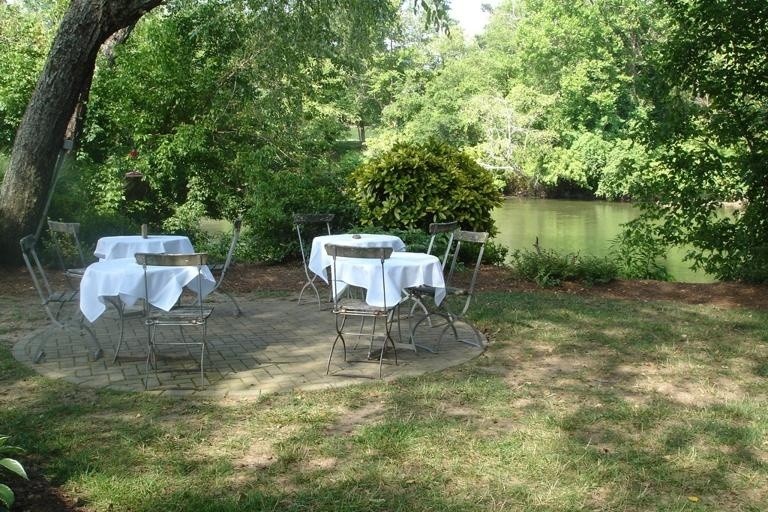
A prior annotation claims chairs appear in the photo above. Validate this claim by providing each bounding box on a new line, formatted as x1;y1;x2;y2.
292;213;491;380
19;218;247;392
